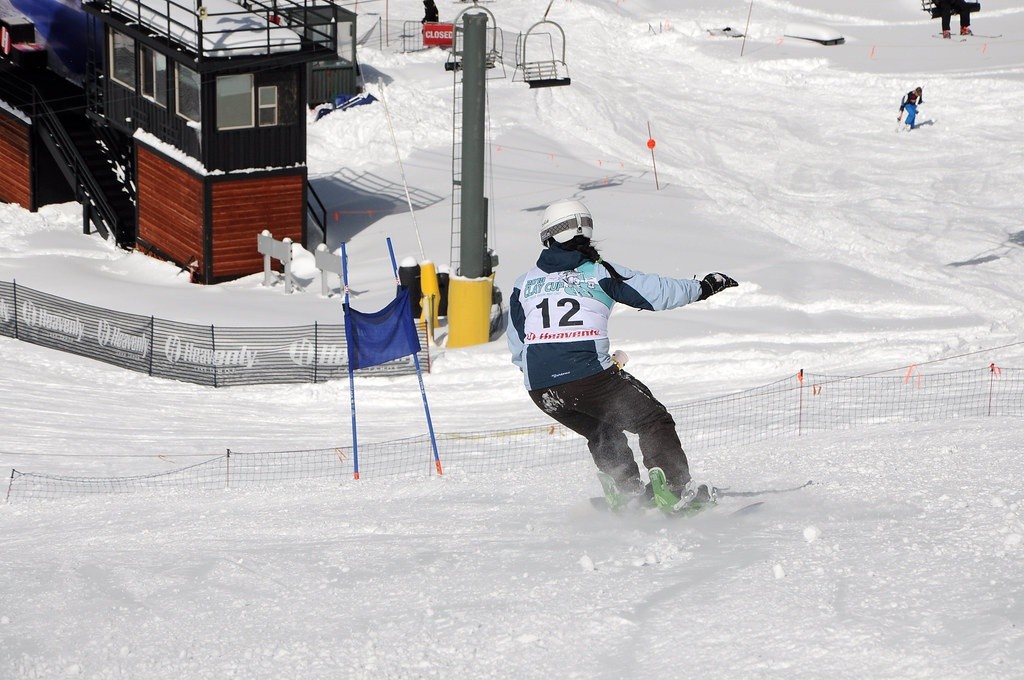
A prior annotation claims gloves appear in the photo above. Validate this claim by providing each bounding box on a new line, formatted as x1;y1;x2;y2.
697;271;739;302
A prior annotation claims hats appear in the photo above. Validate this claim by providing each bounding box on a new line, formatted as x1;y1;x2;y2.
916;88;922;95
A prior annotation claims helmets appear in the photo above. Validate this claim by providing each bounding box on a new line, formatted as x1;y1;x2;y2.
540;200;592;245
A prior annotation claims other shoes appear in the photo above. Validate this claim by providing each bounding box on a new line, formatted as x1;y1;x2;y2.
622;481;654;513
943;30;950;38
657;481;718;514
960;27;970;35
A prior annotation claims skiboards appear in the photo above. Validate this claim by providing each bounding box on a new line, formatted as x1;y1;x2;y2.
932;32;1003;42
721;479;814;517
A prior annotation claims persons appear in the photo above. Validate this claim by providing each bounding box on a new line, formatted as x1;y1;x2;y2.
897;86;922;131
932;0;972;39
419;0;439;34
506;199;738;521
